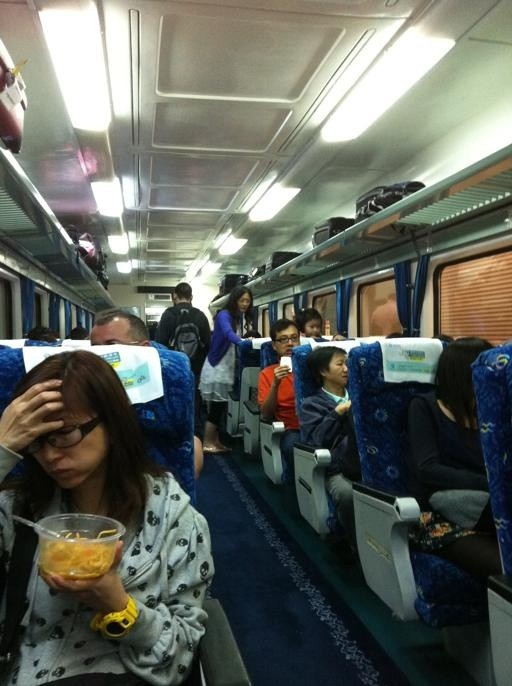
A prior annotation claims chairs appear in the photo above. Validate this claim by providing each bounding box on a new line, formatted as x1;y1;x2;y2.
0;340;250;686
227;335;386;536
471;342;511;686
352;338;450;623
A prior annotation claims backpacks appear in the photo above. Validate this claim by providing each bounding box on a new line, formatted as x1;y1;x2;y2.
166;307;205;363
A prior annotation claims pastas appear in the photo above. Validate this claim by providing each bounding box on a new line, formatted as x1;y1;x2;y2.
39;527;119;581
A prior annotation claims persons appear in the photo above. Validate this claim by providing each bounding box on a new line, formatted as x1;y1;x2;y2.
155;282;211;374
295;308;323;338
306;346;362;549
257;319;348;485
406;337;502;595
198;285;255;453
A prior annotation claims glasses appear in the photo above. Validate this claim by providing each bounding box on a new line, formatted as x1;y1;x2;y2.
272;334;300;343
22;416;105;455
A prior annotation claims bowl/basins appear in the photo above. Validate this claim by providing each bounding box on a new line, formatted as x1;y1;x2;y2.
37;512;127;580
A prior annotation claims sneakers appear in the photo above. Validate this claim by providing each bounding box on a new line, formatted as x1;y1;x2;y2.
202;445;234;455
